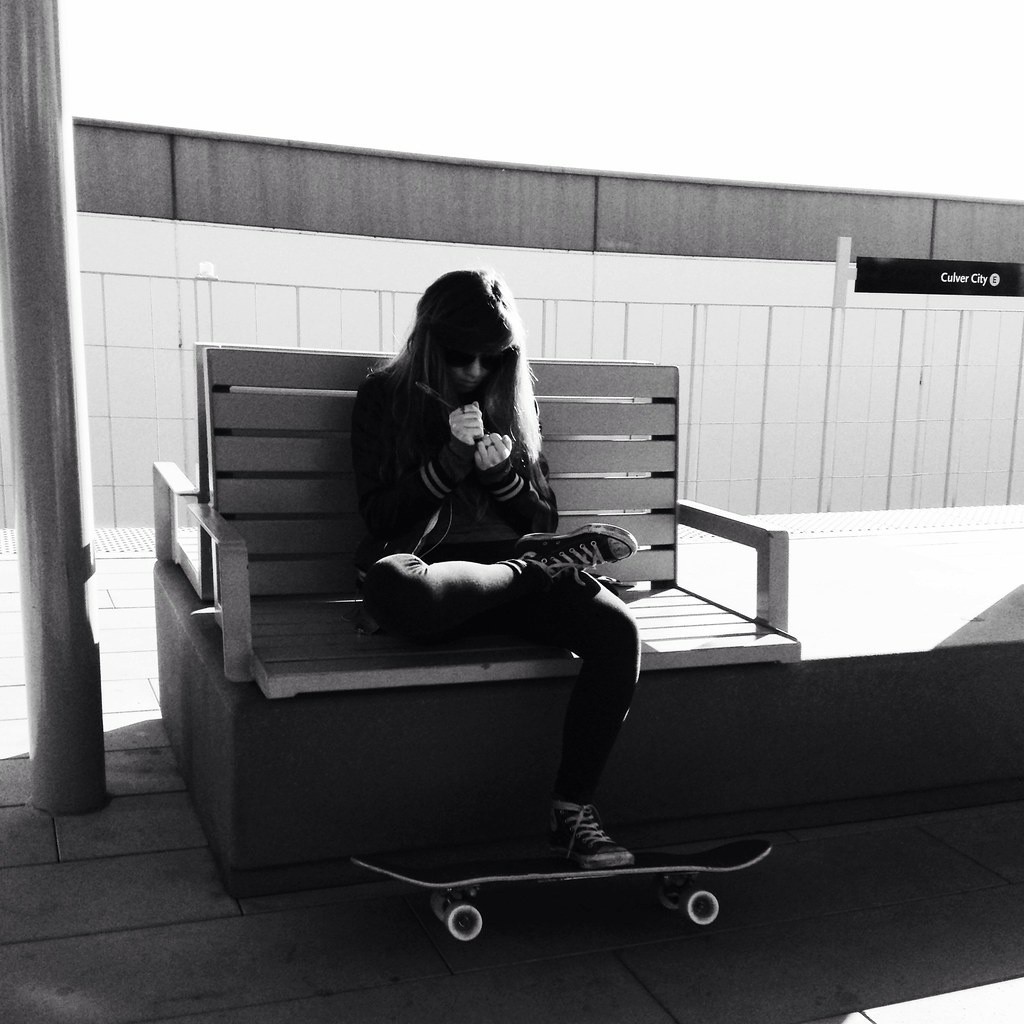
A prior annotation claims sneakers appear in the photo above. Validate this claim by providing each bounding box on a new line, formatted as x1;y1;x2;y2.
550;803;635;869
513;524;633;585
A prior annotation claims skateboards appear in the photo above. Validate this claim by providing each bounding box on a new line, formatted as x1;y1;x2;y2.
349;838;775;941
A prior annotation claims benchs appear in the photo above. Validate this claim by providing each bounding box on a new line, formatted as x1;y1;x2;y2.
151;342;802;698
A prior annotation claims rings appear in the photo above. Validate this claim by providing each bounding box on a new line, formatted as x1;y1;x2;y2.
459;404;465;414
485;442;494;449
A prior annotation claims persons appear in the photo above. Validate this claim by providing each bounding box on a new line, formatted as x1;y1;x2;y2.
349;271;640;868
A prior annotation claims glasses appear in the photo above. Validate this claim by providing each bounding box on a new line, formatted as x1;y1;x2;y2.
443;348;504;371
